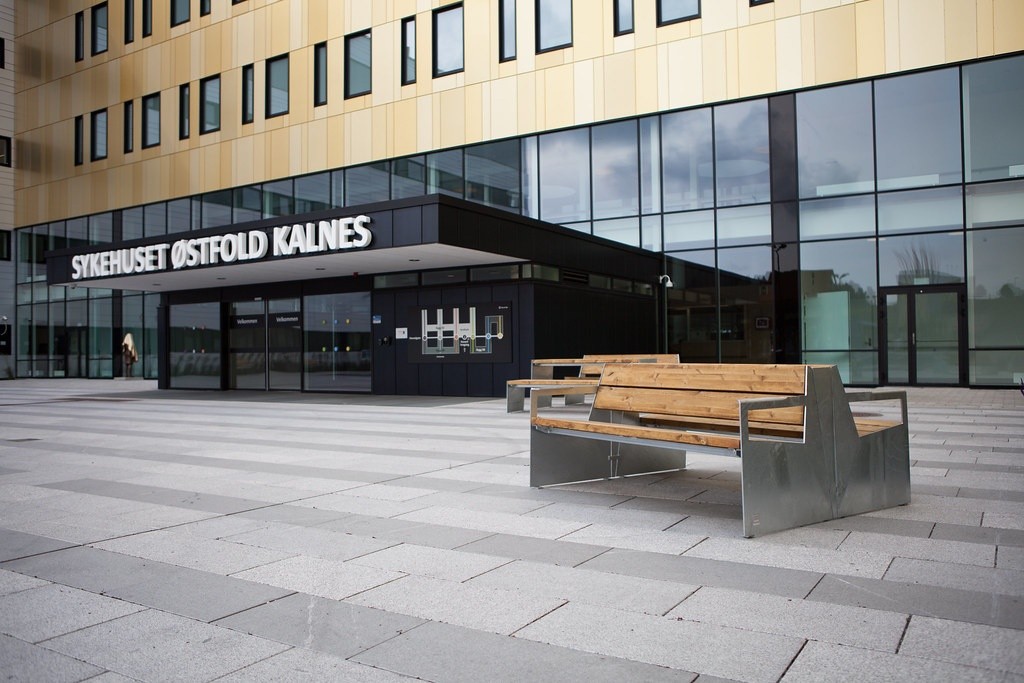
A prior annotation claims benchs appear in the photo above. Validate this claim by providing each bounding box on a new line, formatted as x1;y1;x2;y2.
504;353;913;540
